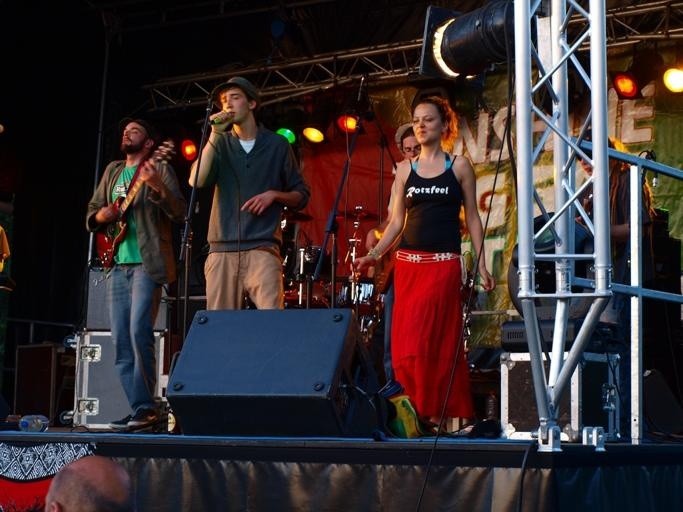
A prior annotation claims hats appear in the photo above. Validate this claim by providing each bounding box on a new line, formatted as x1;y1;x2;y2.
210;75;258;101
117;115;150;133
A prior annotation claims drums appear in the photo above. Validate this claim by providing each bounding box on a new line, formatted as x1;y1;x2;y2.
362;318;387;368
326;277;376;305
298;257;336;279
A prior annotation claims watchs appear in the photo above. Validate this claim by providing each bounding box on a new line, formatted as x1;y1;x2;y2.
155;181;162;192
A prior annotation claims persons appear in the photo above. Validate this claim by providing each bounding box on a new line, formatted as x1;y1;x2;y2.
385;123;463;388
366;193;392;252
45;456;133;512
188;77;310;310
351;97;496;434
0;226;10;272
85;121;186;429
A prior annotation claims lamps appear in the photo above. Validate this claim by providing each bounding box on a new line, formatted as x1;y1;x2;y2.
275;128;295;144
336;115;356;133
180;139;198;160
663;61;683;93
302;127;324;143
613;40;663;100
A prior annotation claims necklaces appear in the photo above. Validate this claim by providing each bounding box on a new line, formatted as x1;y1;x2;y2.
238;134;255;140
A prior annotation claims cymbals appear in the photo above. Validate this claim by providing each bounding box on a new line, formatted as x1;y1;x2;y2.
288;213;311;221
334;210;382;223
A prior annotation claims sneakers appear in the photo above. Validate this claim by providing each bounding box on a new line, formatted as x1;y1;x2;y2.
127;409;158;428
109;414;133;430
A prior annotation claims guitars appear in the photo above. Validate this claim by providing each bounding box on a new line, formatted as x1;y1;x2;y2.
95;140;176;268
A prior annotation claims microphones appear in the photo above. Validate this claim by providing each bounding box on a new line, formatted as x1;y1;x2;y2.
350;102;377;121
209;111;234;127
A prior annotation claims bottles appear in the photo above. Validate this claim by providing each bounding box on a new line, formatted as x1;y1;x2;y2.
486;390;498;420
19;415;50;432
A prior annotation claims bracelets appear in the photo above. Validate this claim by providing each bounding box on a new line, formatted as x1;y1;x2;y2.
209;139;217;151
368;248;380;261
